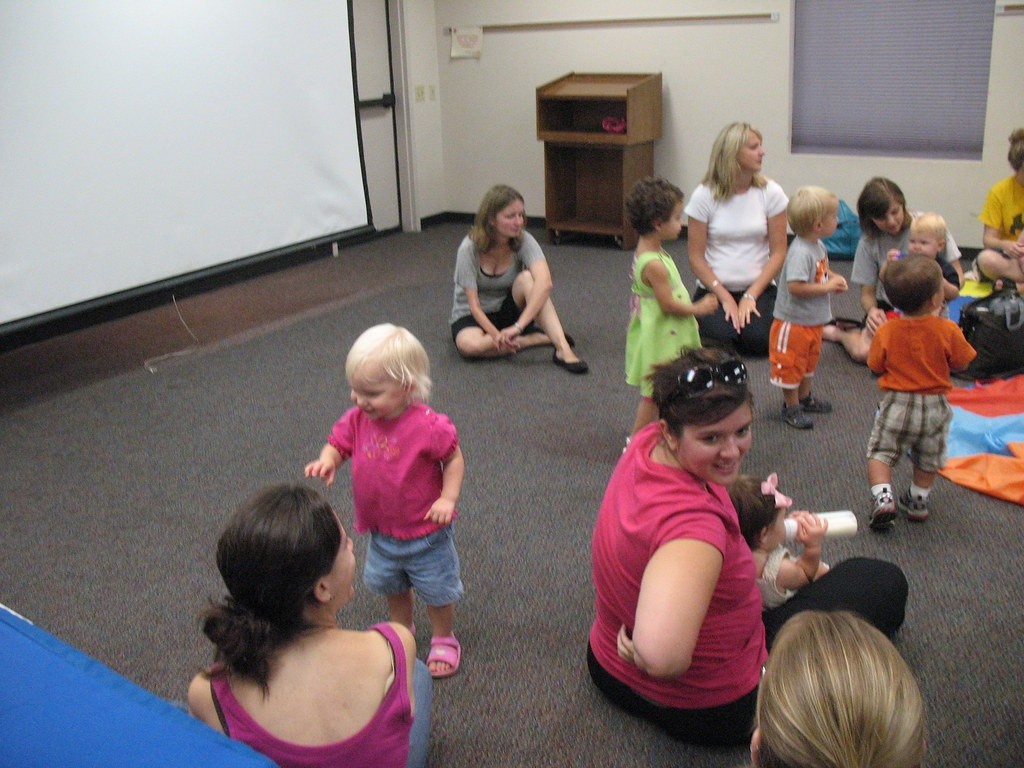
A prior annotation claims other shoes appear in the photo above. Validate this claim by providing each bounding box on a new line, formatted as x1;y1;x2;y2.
552;352;588;374
566;334;575;348
830;317;861;330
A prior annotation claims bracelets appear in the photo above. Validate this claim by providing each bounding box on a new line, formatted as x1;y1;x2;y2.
742;293;757;301
707;280;720;291
514;323;524;331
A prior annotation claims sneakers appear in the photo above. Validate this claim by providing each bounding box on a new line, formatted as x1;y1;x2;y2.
868;488;896;530
898;488;929;521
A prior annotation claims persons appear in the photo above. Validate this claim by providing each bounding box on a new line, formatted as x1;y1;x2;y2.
621;176;718;455
188;482;434;768
1016;230;1024;274
880;212;960;318
865;254;977;529
750;610;929;768
769;186;849;429
683;122;789;356
972;129;1024;293
726;473;829;613
303;323;464;679
450;185;589;374
586;348;910;745
821;178;965;364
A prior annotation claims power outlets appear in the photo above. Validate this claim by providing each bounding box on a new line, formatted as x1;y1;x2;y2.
415;84;424;102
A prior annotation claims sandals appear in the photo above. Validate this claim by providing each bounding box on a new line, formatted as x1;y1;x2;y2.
782;402;813;429
800;392;832;413
426;630;460;679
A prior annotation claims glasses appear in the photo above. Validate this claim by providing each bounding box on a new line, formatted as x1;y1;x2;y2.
666;357;748;403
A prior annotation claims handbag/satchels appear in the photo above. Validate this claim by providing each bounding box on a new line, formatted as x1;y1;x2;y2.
951;288;1024;379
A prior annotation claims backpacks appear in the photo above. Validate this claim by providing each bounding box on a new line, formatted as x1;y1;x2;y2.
822;199;860;260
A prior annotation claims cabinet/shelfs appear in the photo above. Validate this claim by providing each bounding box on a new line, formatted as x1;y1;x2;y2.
536;72;663;250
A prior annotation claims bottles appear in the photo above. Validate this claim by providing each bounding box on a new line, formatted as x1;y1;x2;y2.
783;511;857;542
